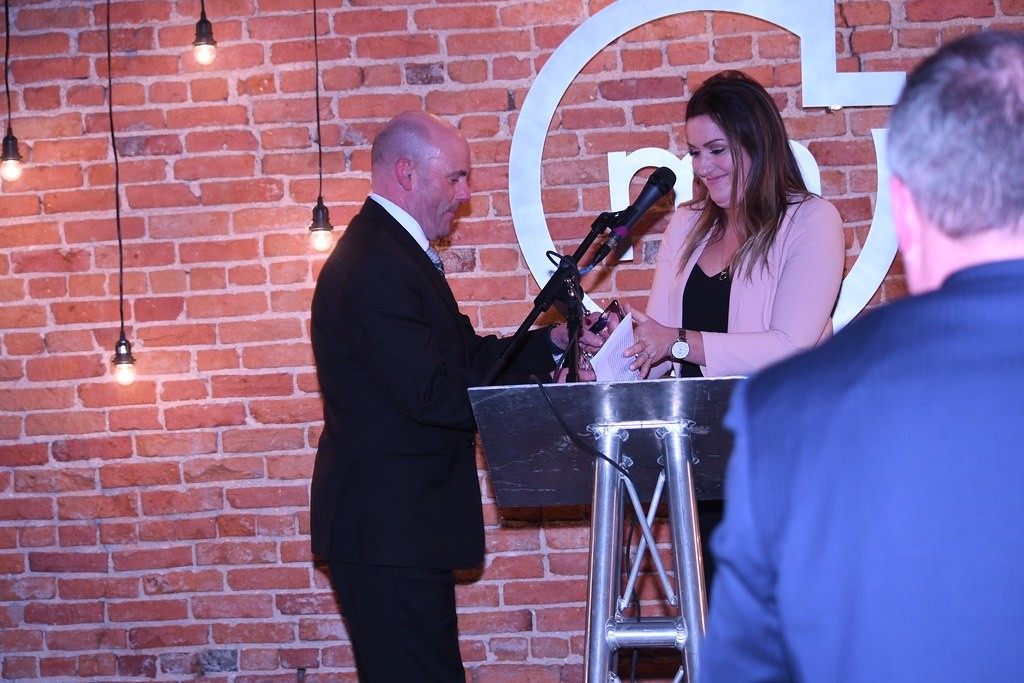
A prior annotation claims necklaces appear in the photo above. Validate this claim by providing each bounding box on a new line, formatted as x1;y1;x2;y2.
719;232;730;282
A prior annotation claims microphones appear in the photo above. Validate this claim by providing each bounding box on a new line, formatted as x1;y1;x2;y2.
591;166;677;264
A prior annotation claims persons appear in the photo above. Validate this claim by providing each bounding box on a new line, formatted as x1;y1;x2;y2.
308;106;620;683
696;26;1021;682
621;68;846;609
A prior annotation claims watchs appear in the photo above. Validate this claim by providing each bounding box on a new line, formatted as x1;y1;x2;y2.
669;327;690;360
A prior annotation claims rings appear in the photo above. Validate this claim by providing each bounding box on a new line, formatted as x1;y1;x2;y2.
642;350;650;359
